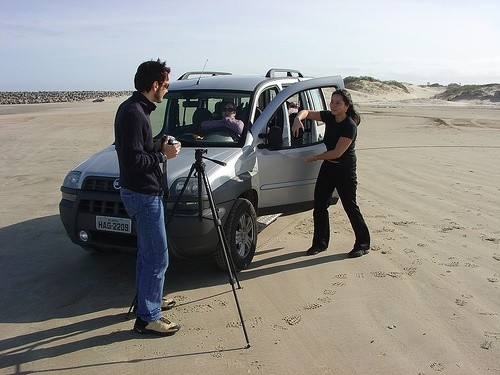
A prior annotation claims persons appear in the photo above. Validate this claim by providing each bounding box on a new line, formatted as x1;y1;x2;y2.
291;89;370;258
114;58;181;336
200;102;245;139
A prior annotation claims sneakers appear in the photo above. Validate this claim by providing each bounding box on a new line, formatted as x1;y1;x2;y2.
134;317;181;336
133;298;176;315
348;244;371;257
306;245;328;256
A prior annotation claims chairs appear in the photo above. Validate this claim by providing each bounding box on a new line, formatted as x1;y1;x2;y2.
212;102;227;120
192;108;215;135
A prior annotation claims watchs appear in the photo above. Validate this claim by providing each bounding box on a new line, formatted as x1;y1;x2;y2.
160;151;167;163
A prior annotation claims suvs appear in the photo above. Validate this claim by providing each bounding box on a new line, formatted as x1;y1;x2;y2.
59;68;344;271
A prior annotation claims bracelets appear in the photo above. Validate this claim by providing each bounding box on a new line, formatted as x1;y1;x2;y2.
311;156;317;162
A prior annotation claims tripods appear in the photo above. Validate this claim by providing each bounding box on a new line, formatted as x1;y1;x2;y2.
126;149;250;348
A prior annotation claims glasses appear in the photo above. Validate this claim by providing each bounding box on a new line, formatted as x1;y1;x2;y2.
161;84;169;89
221;107;237;113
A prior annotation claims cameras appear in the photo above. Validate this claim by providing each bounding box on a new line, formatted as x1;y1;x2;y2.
162;134;180;145
